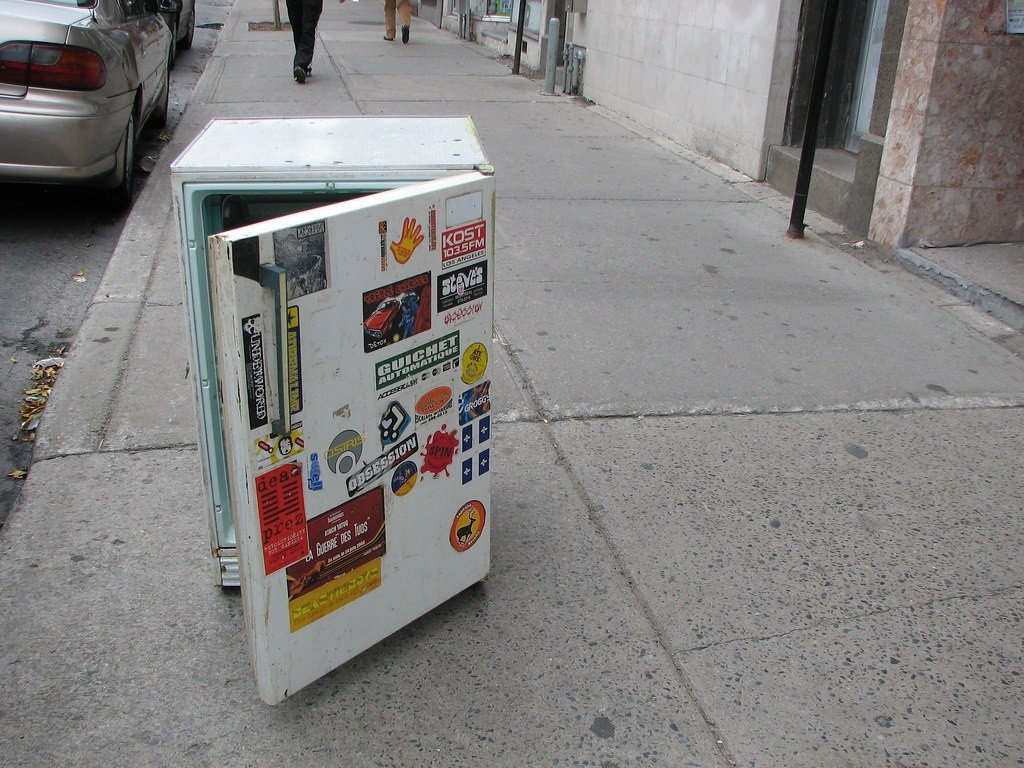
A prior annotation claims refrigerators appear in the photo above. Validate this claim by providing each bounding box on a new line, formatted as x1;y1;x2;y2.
171;114;494;706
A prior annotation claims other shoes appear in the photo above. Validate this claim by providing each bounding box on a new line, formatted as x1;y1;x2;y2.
402;27;409;44
306;63;312;76
384;37;394;41
294;66;306;83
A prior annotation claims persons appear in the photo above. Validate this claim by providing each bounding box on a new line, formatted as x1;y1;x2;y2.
383;0;410;44
286;0;323;83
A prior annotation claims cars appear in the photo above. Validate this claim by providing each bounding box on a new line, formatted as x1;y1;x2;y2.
149;0;197;70
363;297;402;337
0;0;184;215
